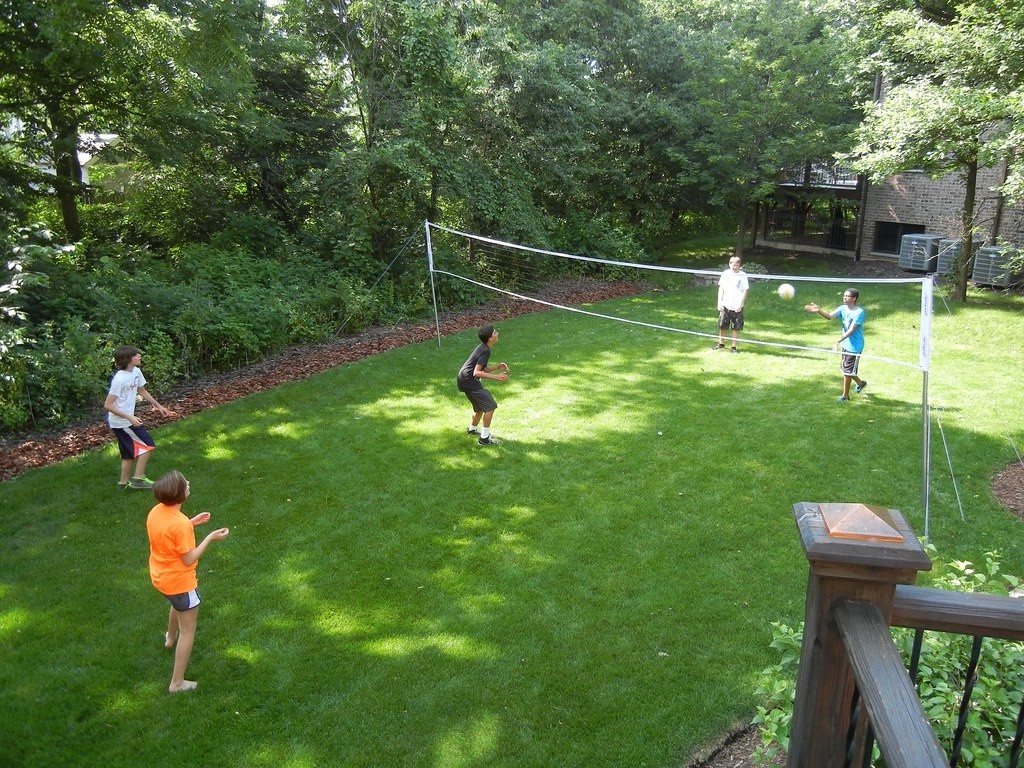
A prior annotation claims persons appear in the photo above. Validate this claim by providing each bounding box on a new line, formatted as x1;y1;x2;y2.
805;288;867;402
104;346;169;489
715;257;749;353
146;471;229;693
457;325;509;447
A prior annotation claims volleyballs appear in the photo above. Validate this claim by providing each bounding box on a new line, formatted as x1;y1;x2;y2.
778;284;795;301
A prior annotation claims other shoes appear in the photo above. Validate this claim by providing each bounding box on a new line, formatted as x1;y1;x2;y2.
731;347;738;352
131;474;156;489
715;344;725;350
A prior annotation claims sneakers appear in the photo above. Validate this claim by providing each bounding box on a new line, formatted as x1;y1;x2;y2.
118;480;131;490
855;380;866;393
836;396;850;402
478;433;503;446
468;428;481;435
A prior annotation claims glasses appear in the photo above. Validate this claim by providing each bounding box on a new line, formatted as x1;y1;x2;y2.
843;295;854;298
